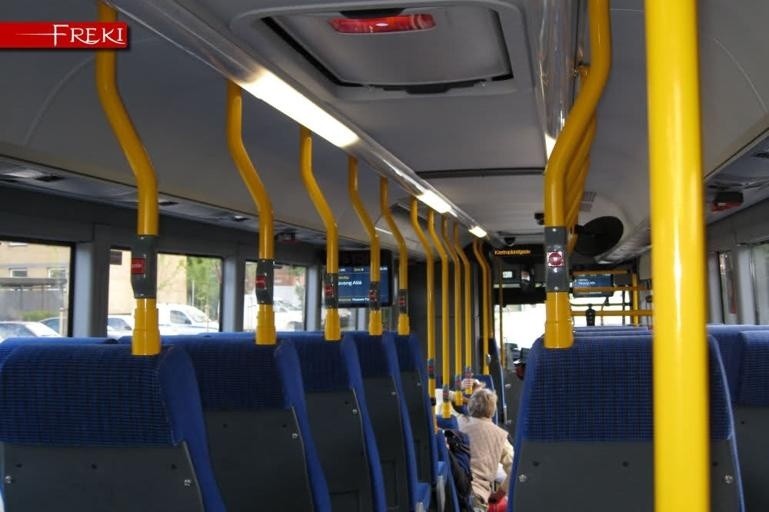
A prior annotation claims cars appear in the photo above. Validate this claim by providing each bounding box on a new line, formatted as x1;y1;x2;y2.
0;304;219;344
241;299;325;332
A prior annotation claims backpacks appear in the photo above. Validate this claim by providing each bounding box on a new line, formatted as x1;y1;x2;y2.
443;429;474;512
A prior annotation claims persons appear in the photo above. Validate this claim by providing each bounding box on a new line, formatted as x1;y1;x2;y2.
457;387;516;512
460;369;486;389
435;386;459;419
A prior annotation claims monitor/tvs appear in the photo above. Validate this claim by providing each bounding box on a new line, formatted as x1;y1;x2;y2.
572;264;614;297
320;249;393;308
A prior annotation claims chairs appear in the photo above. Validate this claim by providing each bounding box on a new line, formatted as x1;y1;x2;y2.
0;324;769;512
